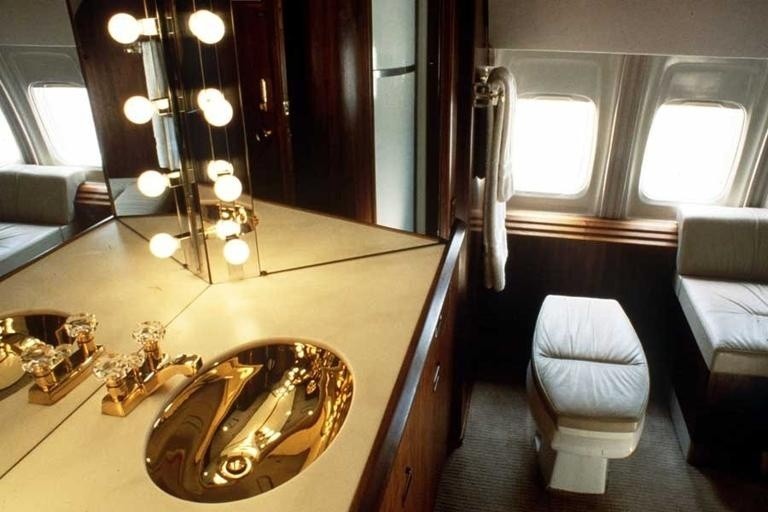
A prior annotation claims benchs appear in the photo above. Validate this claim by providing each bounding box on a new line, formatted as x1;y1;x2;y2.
525;292;650;497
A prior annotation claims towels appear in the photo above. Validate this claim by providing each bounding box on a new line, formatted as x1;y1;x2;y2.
489;66;516;203
483;80;511;291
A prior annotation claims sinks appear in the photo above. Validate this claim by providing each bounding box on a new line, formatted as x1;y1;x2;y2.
143;336;357;508
0;308;76;407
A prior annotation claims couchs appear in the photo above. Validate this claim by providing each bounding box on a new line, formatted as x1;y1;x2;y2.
0;162;89;277
673;206;768;463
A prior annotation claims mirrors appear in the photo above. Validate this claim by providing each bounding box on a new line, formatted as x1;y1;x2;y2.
157;1;446;282
1;1;211;484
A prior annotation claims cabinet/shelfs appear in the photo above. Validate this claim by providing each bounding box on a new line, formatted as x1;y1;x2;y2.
375;242;464;512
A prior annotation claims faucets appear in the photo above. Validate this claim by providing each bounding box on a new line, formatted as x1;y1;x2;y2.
92;322;203;415
0;311;106;405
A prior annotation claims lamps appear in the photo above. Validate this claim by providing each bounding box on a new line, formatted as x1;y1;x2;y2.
139;172;179;196
186;7;227;47
124;95;170;126
149;231;183;260
212;171;242;201
222;237;249;263
109;11;158;48
185;89;234;128
